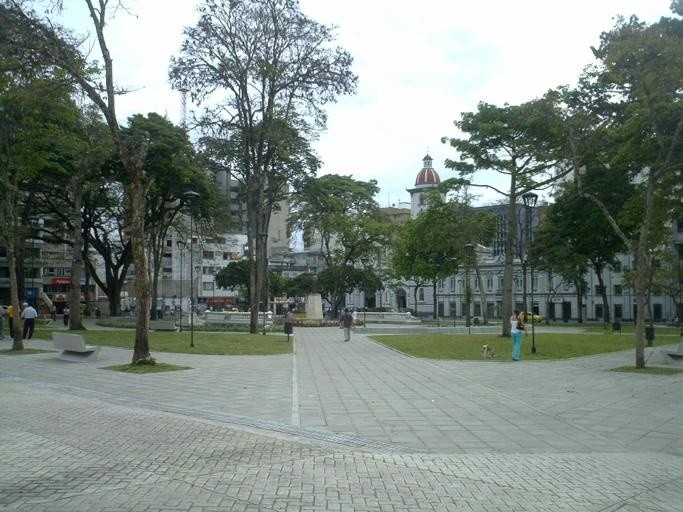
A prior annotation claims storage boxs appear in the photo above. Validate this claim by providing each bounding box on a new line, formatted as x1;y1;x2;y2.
49;330;101;364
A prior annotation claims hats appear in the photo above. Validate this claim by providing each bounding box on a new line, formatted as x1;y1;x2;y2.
519;310;544;323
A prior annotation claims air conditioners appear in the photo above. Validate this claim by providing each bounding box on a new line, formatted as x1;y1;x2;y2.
481;343;496;361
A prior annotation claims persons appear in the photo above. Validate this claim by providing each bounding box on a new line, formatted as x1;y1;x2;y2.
510;309;528;361
5;300;13;341
337;308;353;342
94;305;101;319
49;302;56;322
0;300;6;339
19;301;38;340
350;307;357;328
62;305;69;327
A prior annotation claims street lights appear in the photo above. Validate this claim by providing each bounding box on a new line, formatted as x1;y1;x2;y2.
516;323;524;330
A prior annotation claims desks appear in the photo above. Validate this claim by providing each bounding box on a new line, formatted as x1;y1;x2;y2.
452;243;474;334
522;192;538;348
177;190;202;346
258;233;268;334
